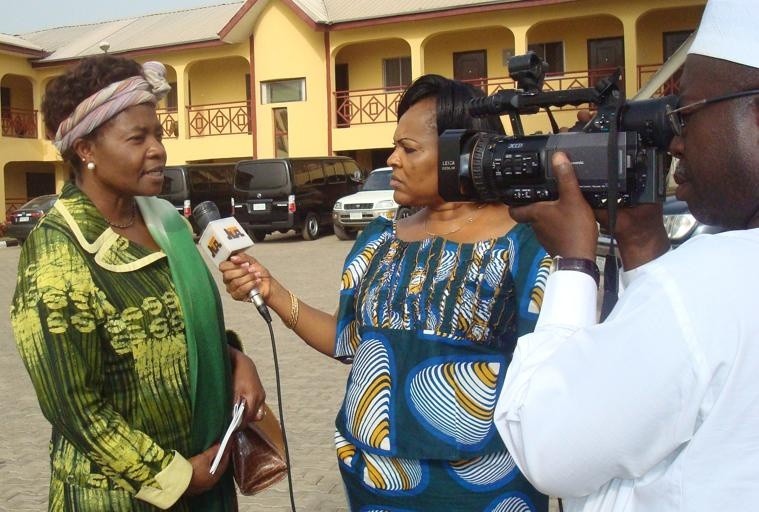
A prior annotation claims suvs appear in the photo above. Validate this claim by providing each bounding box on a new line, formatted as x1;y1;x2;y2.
332;167;415;239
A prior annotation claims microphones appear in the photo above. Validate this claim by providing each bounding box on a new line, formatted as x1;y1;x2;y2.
193;200;273;324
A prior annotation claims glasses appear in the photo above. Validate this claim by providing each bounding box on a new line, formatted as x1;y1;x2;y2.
657;87;756;135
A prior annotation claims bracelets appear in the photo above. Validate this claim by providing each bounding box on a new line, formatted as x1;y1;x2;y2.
280;288;303;332
547;253;602;284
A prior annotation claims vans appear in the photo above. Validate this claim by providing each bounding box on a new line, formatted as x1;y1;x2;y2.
156;162;236;242
231;156;370;242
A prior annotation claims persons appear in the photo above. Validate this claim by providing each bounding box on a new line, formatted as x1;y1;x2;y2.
217;74;559;512
9;55;269;511
487;0;757;510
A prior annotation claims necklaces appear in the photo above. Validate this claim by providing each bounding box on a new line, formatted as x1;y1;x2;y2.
98;202;139;229
424;204;488;237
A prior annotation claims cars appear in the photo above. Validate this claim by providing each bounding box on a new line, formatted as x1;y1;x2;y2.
597;198;725;270
9;195;60;246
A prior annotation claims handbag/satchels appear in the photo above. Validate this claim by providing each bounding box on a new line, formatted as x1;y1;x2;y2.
232;402;290;496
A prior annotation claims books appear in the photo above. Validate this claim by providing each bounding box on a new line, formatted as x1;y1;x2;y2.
209;396;247;476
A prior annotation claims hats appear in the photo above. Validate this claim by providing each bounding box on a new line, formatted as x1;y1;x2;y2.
686;2;759;72
